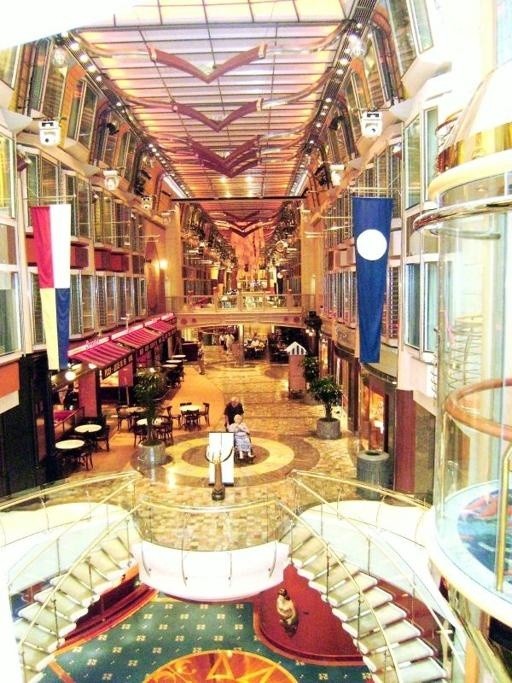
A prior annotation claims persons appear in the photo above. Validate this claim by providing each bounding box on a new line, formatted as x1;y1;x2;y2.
224;397;244;427
197;342;205;375
276;588;299;637
219;332;235;353
228;414;255;459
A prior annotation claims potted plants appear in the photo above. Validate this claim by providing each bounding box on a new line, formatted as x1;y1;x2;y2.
295;358;348;440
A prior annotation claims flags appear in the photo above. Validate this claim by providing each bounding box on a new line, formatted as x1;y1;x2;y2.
28;204;75;370
352;196;393;364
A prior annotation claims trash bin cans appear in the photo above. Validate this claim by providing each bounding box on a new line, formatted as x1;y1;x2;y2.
357;449;391;500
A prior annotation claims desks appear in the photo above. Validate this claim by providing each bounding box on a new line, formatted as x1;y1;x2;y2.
56;337;213;479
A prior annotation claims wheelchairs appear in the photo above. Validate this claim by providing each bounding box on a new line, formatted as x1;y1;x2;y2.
226;425;254;464
